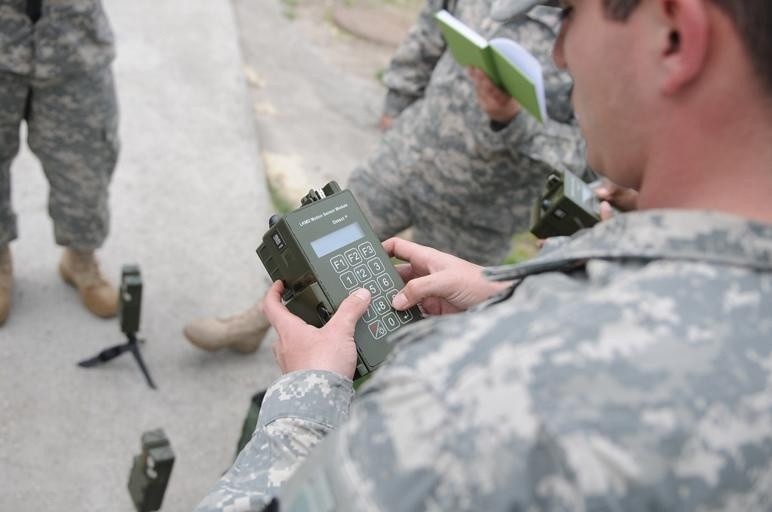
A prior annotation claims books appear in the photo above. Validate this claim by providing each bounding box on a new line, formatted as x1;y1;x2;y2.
432;9;549;125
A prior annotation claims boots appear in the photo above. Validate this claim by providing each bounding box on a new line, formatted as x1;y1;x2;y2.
0;244;13;322
60;247;119;317
183;294;271;354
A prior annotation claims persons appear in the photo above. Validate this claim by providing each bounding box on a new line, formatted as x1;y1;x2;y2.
183;0;588;382
192;0;769;510
533;178;643;248
0;0;125;329
